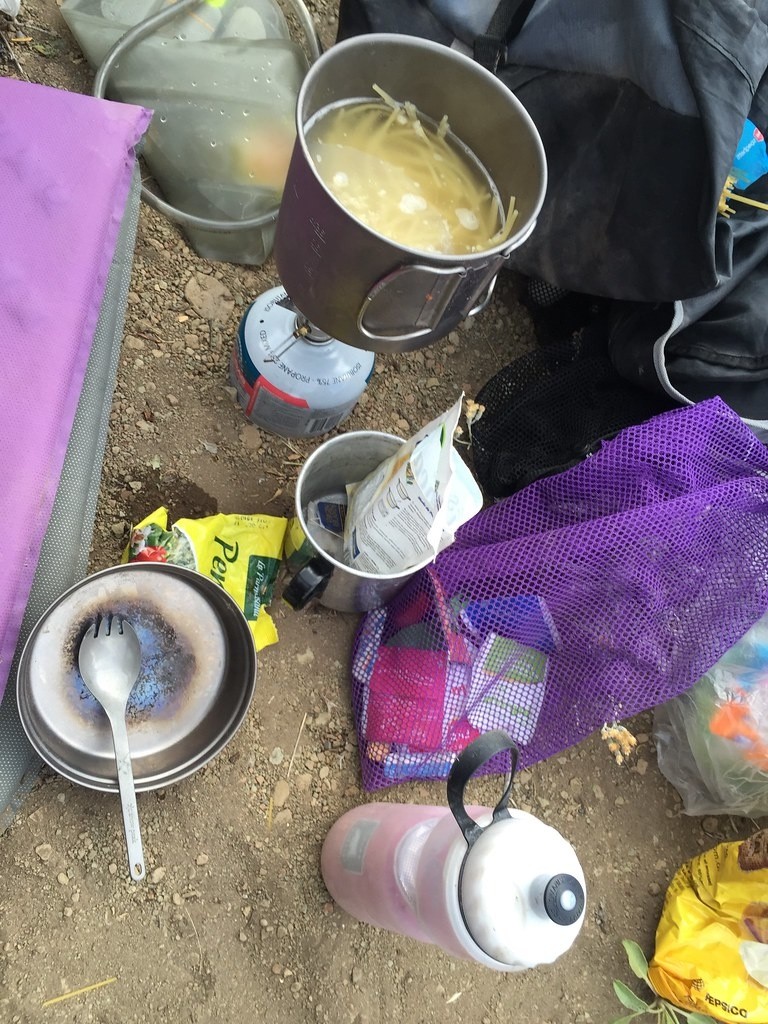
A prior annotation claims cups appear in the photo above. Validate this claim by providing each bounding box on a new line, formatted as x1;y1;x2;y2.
282;432;433;612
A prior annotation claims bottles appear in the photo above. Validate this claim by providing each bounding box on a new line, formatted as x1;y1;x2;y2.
320;802;586;973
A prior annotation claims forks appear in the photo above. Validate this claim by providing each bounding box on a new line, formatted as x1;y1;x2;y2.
79;618;141;881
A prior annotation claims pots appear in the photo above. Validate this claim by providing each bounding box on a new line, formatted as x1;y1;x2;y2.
273;32;550;351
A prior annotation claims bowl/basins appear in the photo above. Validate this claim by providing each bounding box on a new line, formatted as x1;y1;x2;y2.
18;562;255;795
